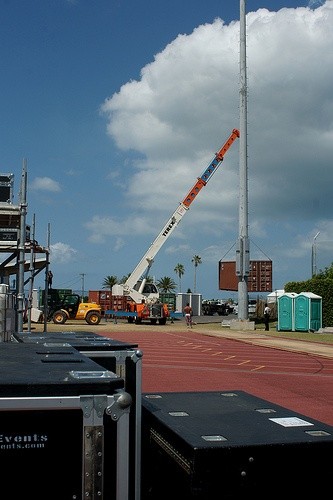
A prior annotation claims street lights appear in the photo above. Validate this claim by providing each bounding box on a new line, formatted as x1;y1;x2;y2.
314;232;320;277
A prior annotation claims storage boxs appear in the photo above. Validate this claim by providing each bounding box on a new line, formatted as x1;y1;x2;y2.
0;173;31;241
219;260;272;293
0;284;333;500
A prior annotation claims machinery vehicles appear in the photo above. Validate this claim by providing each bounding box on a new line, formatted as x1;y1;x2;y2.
48;295;104;324
102;127;240;324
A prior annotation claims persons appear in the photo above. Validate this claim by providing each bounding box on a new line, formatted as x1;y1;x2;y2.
126;302;131;312
183;302;194;329
263;303;272;331
